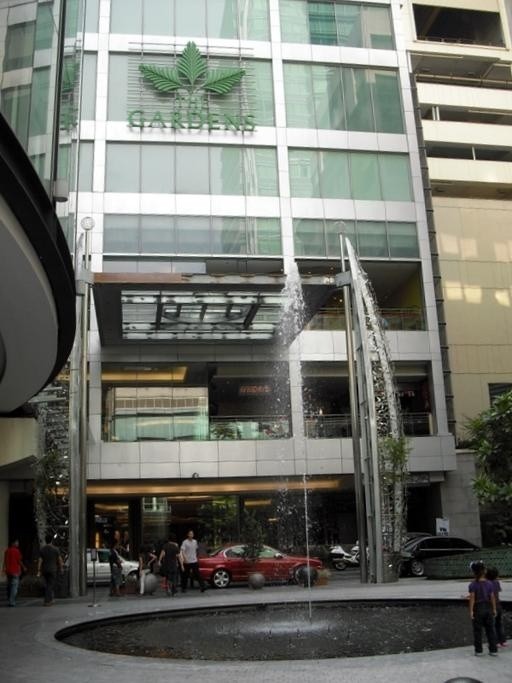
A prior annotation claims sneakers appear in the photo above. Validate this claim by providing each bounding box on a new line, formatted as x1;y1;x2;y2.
474;641;508;656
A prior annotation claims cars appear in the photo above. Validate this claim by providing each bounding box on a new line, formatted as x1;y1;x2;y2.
86;548;139;583
198;542;323;589
405;532;480;577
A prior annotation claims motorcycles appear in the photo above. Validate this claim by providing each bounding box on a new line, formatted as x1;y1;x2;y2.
330;540;360;571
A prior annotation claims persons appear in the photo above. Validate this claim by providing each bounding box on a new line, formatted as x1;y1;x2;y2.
36;535;64;606
469;560;508;657
109;529;207;597
3;538;28;606
